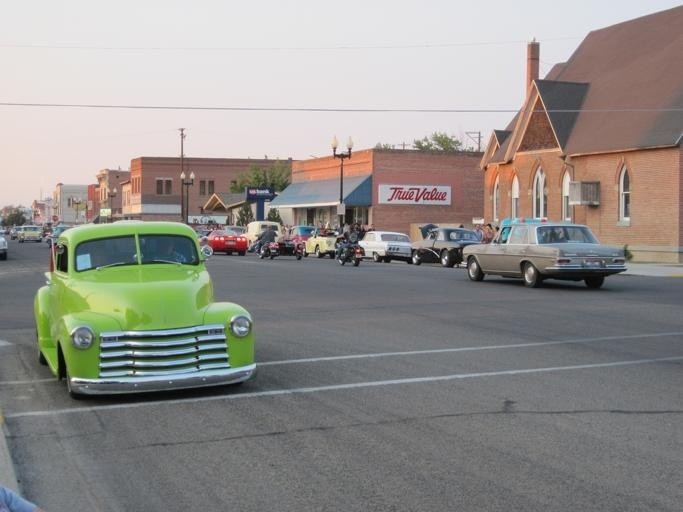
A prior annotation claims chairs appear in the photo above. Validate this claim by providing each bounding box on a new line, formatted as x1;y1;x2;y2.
78;241;120;270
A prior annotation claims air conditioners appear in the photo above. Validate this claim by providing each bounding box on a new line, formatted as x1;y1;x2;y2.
568;181;600;207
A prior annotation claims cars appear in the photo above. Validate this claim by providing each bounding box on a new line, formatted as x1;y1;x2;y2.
30;217;259;403
0;222;69;260
193;219;414;269
406;226;484;269
459;220;629;293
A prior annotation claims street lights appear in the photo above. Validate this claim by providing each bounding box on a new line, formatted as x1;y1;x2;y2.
105;182;118;222
72;196;83;218
327;133;354;230
178;170;196;225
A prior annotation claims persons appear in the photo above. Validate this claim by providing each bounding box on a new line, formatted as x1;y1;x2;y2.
254;221;375;260
459;224;499;244
